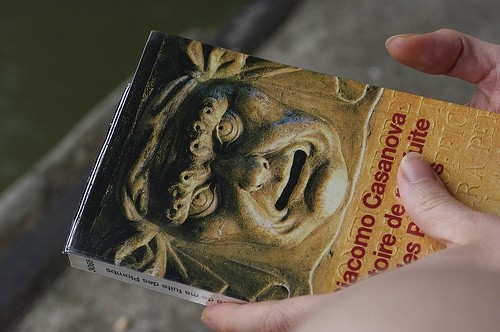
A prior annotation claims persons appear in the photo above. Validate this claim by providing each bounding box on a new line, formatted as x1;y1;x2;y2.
113;40;347;281
199;28;500;332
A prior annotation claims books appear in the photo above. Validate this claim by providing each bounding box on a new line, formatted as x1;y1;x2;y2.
62;29;500;308
